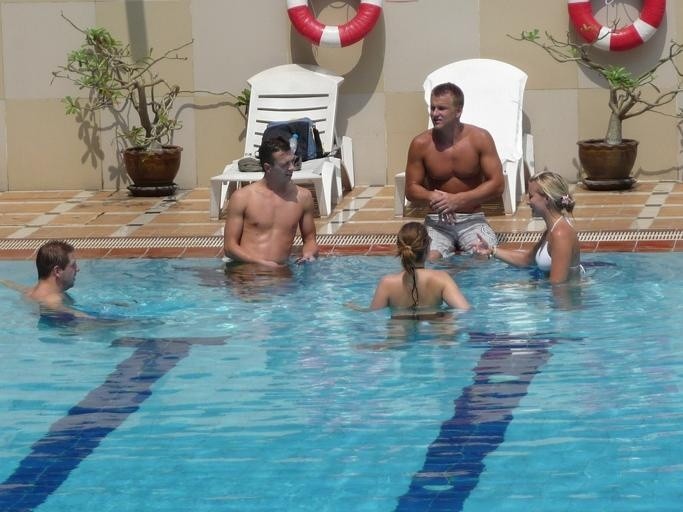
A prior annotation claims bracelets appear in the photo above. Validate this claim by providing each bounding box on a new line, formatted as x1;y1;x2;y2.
490;245;496;258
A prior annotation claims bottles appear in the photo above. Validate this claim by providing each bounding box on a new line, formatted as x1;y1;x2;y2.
289;134;298;154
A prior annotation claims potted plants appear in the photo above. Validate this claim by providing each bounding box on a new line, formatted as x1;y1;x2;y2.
48;8;251;196
505;16;683;191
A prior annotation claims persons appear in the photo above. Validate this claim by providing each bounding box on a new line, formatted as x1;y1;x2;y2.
343;223;471;354
405;83;506;260
468;170;579;315
223;138;317;279
0;240;143;330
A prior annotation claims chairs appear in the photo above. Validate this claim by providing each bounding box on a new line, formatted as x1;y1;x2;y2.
209;62;355;218
391;58;535;218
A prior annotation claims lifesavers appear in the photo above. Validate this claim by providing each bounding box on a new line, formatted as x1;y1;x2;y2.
287;1;382;46
567;1;666;51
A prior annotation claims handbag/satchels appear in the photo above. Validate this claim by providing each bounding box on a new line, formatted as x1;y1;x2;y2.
261;117;325;160
